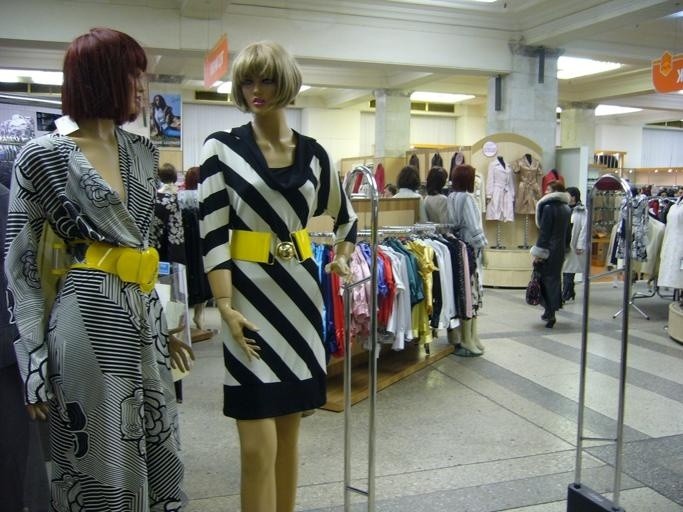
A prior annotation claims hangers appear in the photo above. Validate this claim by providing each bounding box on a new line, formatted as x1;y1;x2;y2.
310;224;452;245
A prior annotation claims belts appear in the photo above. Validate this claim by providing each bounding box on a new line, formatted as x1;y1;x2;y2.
68;239;161;295
228;226;315;264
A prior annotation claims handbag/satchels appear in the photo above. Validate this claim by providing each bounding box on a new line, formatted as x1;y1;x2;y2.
526;281;542;306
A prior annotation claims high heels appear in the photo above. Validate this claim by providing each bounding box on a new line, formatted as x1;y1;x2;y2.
540;308;556;328
563;288;575;300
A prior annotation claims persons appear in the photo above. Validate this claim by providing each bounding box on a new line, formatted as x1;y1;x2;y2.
2;28;196;512
197;39;356;512
184;165;212;329
149;94;181;141
391;166;428;223
523;181;571;328
559;186;586;303
382;182;395;197
158;162;177;193
485;156;515;222
541;168;566;192
513;153;544;213
613;187;648;262
423;167;449;233
657;191;668;198
447;165;488;354
